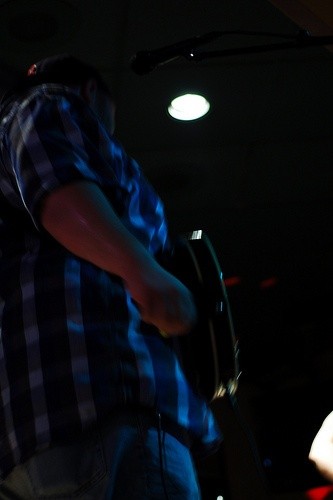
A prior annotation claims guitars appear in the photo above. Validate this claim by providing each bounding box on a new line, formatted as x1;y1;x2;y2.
176;229;239;403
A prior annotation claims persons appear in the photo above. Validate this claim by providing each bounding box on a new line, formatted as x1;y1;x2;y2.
0;51;224;499
